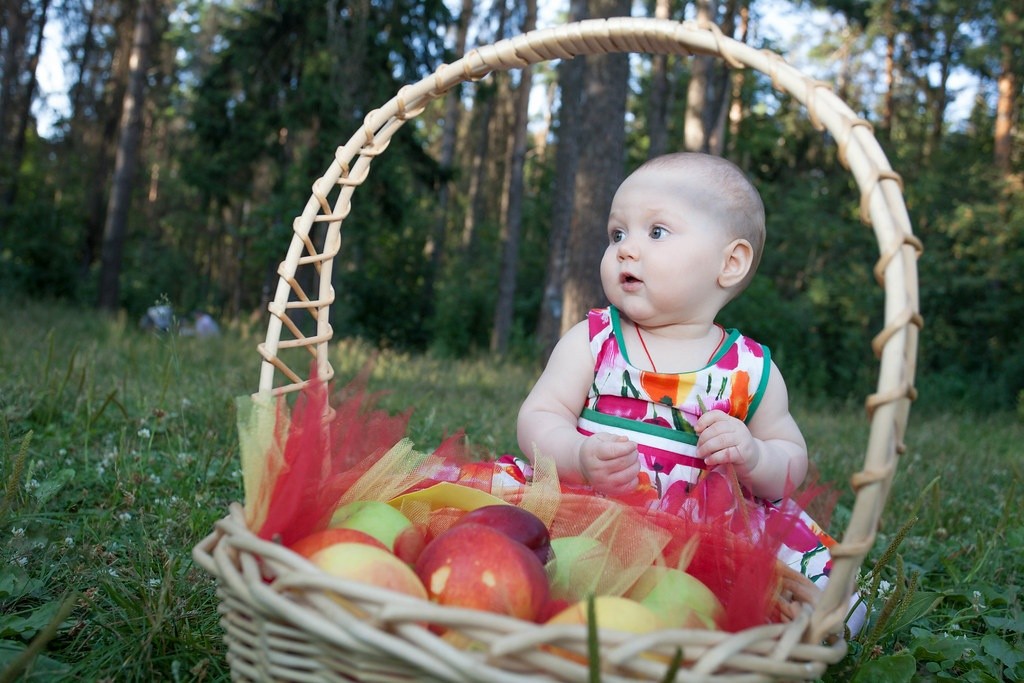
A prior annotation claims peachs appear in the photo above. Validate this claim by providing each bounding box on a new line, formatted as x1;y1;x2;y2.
261;504;731;676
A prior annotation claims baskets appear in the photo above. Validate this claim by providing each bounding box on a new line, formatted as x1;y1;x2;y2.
188;18;922;683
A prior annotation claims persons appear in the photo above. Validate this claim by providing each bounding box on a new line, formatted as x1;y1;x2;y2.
449;152;866;643
137;301;222;340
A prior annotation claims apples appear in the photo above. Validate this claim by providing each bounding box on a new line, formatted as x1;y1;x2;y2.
326;500;422;569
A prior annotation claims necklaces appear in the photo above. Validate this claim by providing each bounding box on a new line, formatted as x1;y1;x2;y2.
633;320;725;375
